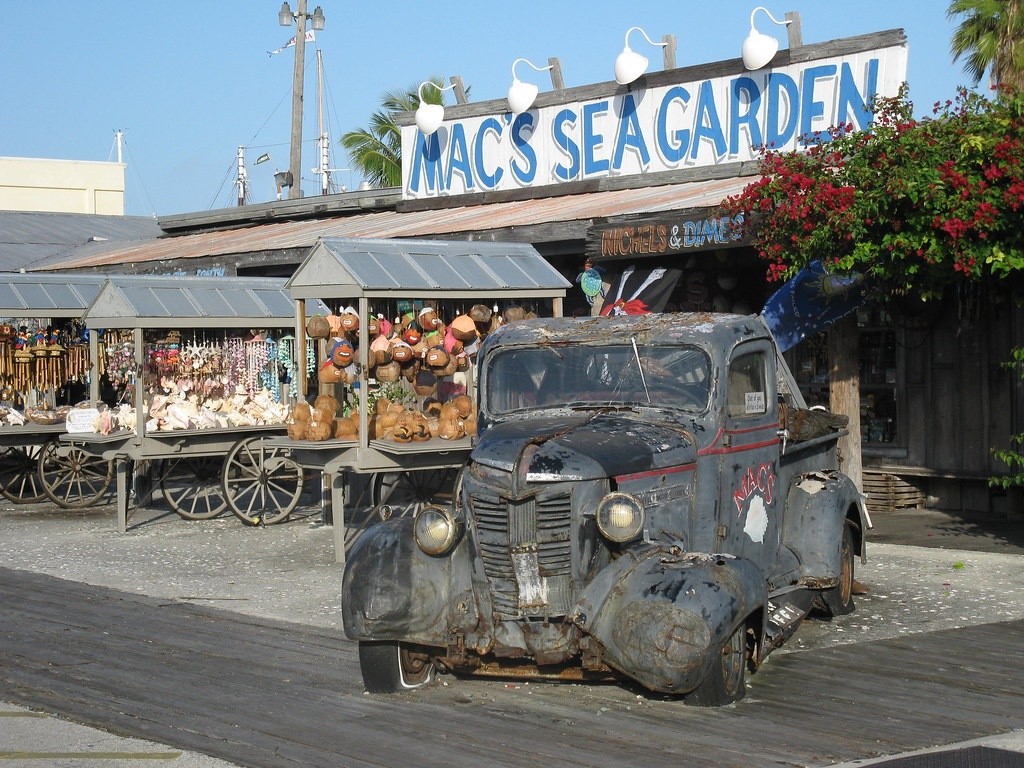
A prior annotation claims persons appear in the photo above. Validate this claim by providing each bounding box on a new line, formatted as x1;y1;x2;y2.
53;370;118;408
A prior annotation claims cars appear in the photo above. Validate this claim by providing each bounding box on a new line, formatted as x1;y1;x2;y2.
342;312;867;706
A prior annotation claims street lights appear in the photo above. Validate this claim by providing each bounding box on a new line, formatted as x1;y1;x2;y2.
278;2;325;199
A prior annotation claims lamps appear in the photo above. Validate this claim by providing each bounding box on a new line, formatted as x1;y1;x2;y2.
508;59;554;115
414;81;456;136
614;27;669;85
274;170;293;189
742;6;793;71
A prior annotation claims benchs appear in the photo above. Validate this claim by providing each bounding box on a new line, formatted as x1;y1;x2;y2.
863;465;1024;522
